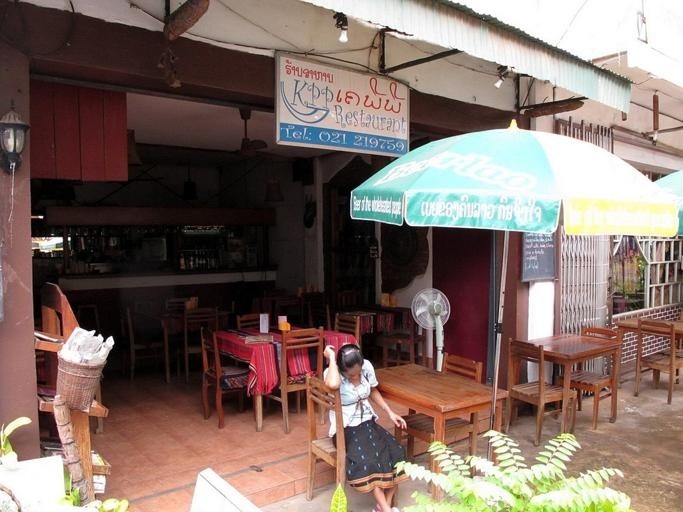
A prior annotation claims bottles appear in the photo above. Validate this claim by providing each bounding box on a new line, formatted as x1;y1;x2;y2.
179;249;217;272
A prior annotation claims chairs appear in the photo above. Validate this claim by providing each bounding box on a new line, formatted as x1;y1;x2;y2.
554;325;623;423
306;376;398;508
395;352;483;478
504;337;577;447
634;311;683;405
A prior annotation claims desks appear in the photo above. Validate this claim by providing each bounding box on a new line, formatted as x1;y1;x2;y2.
616;319;683;388
375;364;509;501
513;334;620;433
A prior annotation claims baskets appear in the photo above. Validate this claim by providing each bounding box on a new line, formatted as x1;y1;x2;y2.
56;351;107;410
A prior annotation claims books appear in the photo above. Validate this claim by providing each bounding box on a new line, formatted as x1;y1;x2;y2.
245;335;274;345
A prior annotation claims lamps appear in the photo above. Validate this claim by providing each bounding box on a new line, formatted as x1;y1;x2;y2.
494;65;509;89
333;12;349;43
0;99;30;175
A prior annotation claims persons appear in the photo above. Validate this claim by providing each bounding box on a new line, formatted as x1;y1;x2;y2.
323;343;407;512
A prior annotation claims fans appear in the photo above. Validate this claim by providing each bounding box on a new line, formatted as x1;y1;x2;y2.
411;287;451;371
231;105;269;162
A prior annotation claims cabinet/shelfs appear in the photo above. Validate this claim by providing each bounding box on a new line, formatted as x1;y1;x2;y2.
35;280;109;440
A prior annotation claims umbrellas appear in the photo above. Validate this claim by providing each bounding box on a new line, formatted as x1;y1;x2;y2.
652;170;683;237
350;119;679;462
